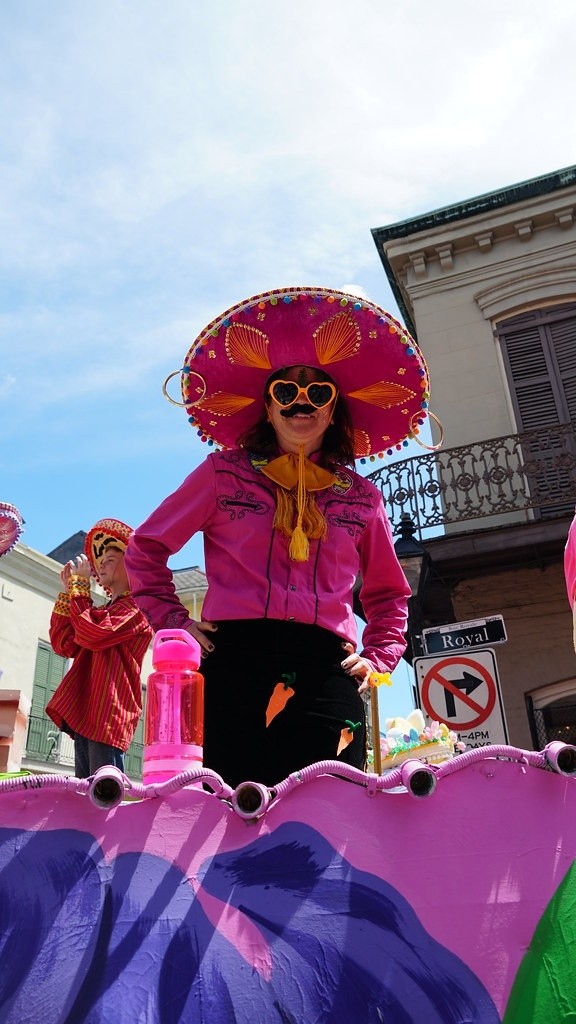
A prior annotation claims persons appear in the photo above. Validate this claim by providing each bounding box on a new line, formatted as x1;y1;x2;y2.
125;288;427;808
563;516;576;648
44;518;153;779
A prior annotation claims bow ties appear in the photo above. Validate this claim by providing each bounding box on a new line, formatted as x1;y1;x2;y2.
261;452;338;491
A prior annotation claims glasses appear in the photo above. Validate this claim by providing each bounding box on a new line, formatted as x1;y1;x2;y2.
268;379;337;411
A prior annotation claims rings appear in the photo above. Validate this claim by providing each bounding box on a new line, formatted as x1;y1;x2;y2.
367;672;391;687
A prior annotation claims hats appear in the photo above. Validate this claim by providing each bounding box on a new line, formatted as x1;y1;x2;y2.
163;286;445;465
84;518;135;598
0;502;25;558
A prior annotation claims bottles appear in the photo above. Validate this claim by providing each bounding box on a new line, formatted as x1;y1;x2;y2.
142;629;205;787
